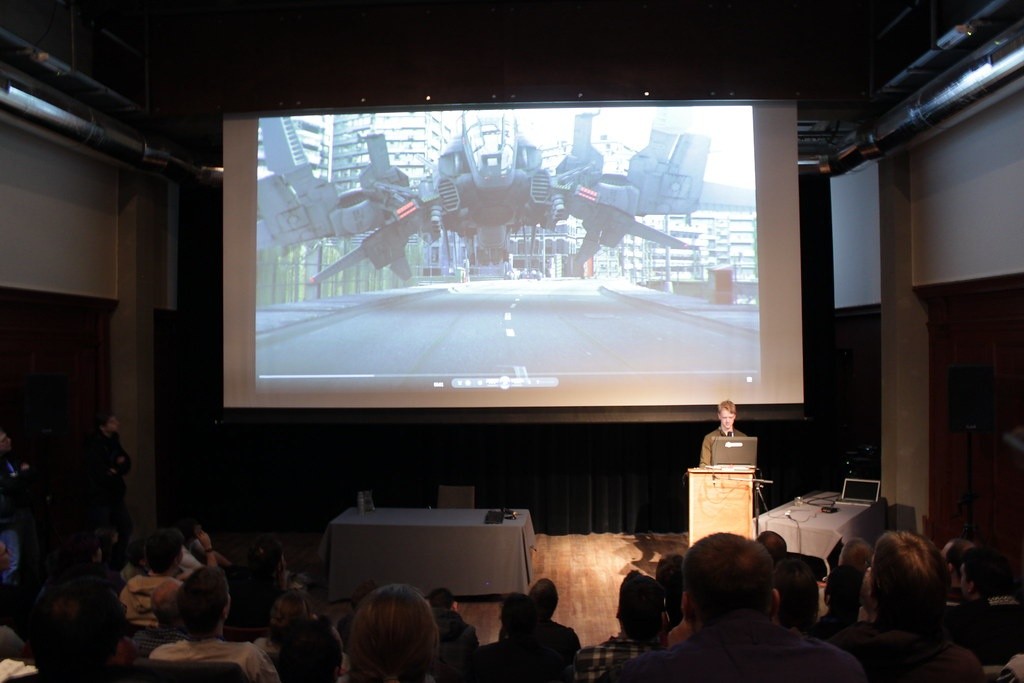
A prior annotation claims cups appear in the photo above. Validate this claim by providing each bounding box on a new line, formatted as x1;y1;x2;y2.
795;497;803;506
359;492;371;514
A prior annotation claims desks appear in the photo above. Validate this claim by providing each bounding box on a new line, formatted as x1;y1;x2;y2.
316;507;539;606
753;489;888;586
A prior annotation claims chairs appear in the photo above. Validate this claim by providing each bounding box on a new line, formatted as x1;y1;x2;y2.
19;616;274;683
435;486;477;509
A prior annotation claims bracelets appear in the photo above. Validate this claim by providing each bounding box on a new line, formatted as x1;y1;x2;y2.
206;548;213;553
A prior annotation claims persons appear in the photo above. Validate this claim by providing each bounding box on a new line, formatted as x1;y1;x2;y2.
0;519;1024;682
0;430;38;569
83;412;133;565
700;400;748;467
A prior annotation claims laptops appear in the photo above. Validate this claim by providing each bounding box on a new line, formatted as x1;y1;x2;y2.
833;478;880;507
709;435;757;469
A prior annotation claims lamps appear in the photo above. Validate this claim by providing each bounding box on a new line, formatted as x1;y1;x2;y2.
818;144;883;179
205;143;224;172
